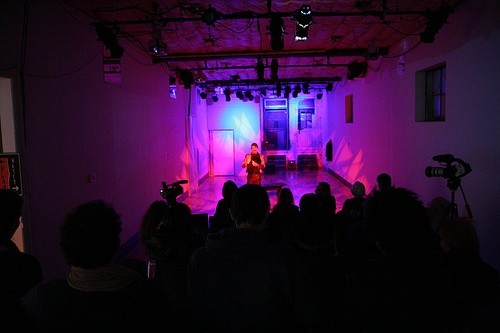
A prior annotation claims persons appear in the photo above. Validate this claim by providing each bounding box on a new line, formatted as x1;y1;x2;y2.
0;172;500;332
241;142;266;184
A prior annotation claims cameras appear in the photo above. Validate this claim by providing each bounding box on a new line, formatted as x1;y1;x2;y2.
425;154;458;178
160;180;188;199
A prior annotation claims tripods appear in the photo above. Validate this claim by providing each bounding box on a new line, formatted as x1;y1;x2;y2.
446;178;461;218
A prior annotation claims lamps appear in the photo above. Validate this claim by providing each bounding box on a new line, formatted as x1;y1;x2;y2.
98;30;126;59
256;61;265;81
347;62;365;81
224;87;231;102
294;4;311;41
295;84;300;93
244;89;251;99
326;82;332;91
293;91;298;99
270;15;285;51
235;89;242;98
284;85;291;97
303;83;309;94
276;83;282;97
316;93;323;99
270;59;279;80
199;91;207;99
419;5;449;44
181;71;192;90
212;95;218;102
